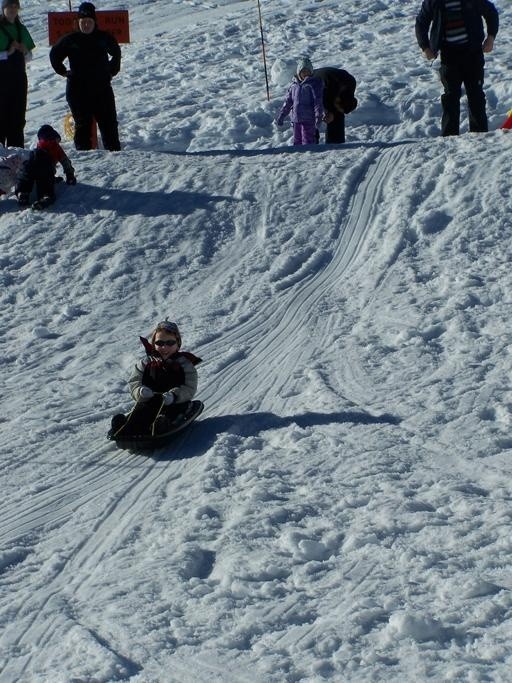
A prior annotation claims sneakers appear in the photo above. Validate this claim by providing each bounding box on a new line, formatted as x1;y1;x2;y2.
17;192;50;210
111;414;127;432
152;415;169;435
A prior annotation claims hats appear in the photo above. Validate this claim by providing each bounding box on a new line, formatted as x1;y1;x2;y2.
152;320;181;347
38;124;61;141
78;2;96;21
297;58;313;81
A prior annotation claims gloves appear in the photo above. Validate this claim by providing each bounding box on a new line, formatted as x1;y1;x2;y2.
316;117;321;125
54;171;77;186
277;116;283;125
140;387;154;398
162;392;174;406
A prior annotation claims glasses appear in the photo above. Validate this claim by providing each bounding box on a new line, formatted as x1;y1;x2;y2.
301;70;311;75
154;338;177;346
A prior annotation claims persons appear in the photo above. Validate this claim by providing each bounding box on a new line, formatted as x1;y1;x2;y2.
415;0;499;137
0;0;122;212
111;321;203;433
277;58;357;146
